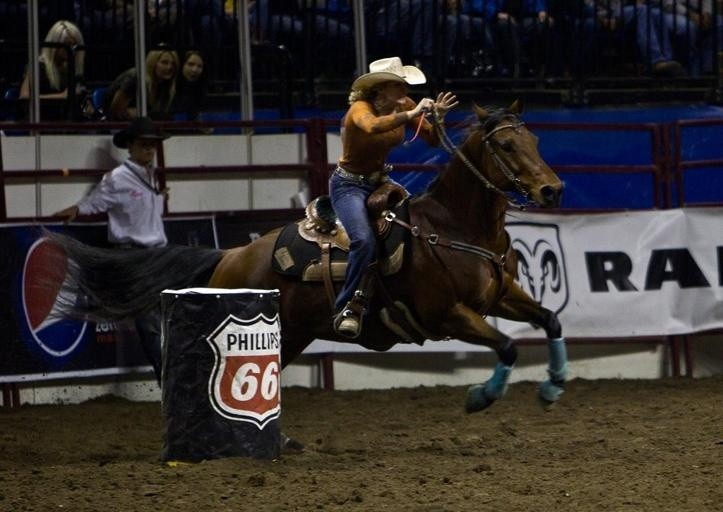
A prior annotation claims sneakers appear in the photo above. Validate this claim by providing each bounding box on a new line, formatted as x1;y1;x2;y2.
337;317;359;337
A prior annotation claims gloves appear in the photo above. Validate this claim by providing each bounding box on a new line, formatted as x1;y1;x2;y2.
406;98;433;119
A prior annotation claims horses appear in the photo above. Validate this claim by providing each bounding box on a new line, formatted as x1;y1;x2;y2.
29;98;568;413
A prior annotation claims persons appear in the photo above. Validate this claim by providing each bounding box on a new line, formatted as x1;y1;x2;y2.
413;1;723;101
17;18;218;137
328;55;461;337
52;115;175;390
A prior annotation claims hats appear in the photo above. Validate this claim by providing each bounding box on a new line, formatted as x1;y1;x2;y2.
113;117;172;148
350;57;427;91
